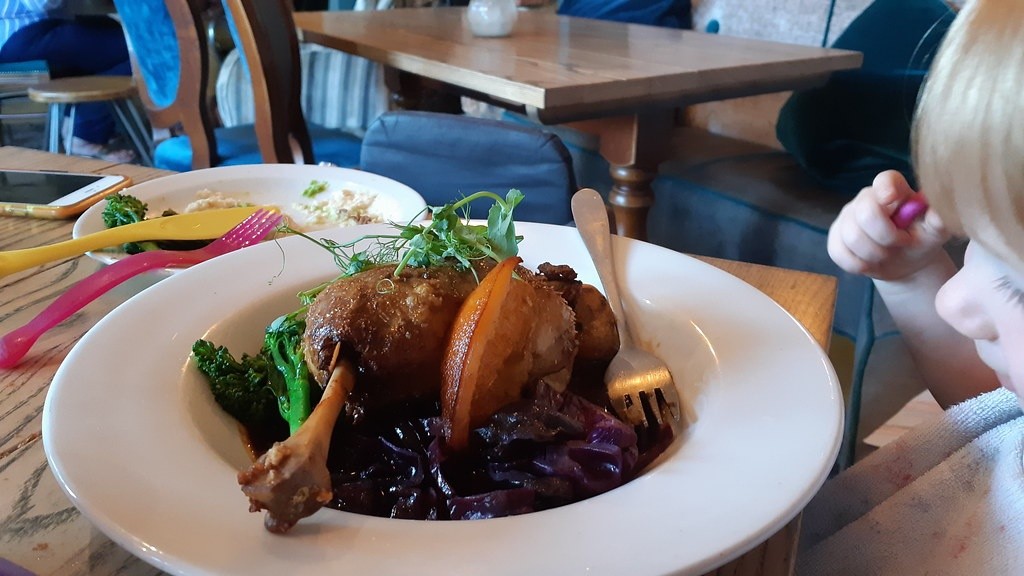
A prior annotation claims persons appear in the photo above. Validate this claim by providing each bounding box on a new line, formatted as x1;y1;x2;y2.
825;0;1024;413
0;0;135;164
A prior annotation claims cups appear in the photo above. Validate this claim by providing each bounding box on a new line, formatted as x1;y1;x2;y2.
467;0;518;38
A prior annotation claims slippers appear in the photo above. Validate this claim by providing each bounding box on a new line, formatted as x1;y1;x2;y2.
70;141;136;163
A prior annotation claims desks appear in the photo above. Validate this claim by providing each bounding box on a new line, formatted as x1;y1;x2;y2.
291;1;868;239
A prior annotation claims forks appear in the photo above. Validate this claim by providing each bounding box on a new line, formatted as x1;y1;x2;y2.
570;188;681;426
0;209;284;371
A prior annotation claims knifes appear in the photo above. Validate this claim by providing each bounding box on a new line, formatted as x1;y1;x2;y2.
0;205;281;278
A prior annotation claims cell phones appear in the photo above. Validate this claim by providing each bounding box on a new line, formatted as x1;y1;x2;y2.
0;168;129;219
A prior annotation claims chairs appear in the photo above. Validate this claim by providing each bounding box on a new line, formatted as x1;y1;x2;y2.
0;0;1024;576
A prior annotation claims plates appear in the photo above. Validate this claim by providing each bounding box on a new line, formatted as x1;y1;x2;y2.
72;160;427;279
40;218;848;576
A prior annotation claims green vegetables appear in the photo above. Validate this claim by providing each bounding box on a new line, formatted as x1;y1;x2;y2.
192;313;311;440
273;181;525;312
102;193;215;254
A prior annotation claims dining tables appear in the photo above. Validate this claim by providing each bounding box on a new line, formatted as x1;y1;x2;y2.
0;140;847;576
